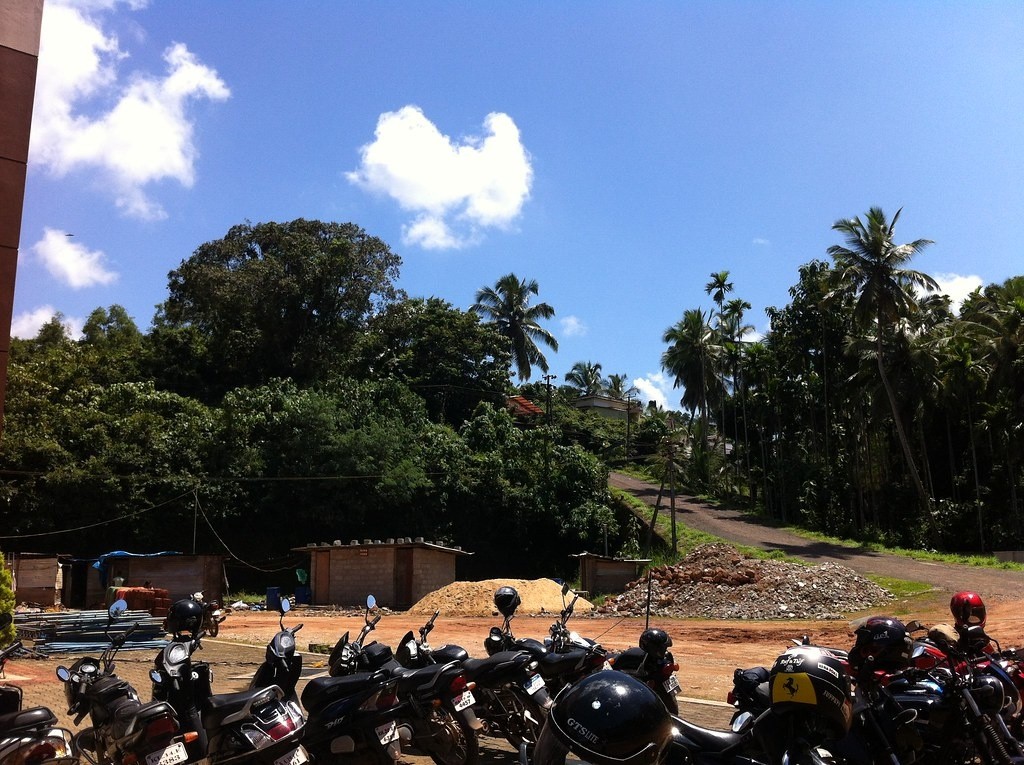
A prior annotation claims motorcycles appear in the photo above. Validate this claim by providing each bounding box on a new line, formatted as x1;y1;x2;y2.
328;595;488;765
543;583;681;718
484;607;606;702
56;599;198;765
533;625;1024;765
191;590;219;637
395;609;554;754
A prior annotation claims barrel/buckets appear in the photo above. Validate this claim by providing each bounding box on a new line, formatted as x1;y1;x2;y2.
295;584;312;605
267;587;280;610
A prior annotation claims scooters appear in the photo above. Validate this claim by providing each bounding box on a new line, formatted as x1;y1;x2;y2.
0;613;80;765
149;632;307;765
248;594;401;765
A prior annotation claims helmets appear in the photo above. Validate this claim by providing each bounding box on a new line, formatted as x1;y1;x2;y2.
854;617;913;662
639;629;672;659
769;646;853;740
967;674;1004;724
495;587;521;617
950;591;986;628
168;600;203;632
547;670;674;765
